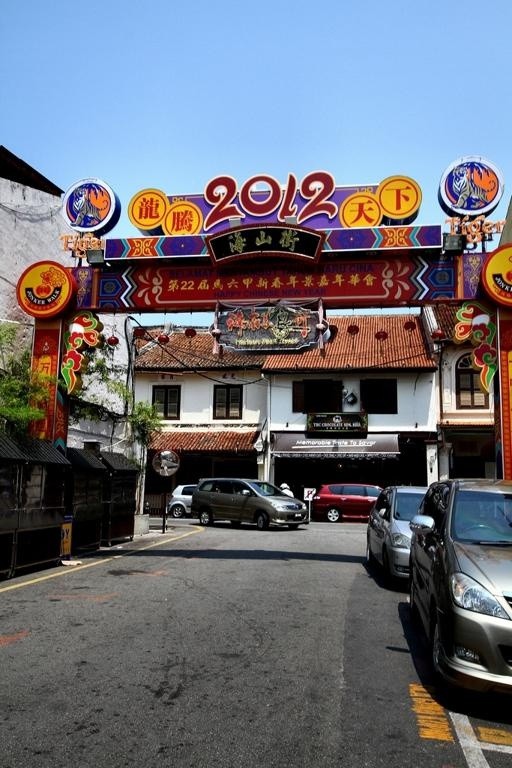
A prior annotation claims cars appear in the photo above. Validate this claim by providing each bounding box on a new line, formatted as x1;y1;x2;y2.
367;486;429;578
168;485;197;518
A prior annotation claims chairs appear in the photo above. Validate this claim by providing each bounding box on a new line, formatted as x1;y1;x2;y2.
458;502;480;523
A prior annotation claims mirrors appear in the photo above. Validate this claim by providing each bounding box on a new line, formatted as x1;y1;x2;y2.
152;449;181;477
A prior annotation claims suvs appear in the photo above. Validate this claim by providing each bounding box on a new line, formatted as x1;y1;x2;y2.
408;478;512;692
312;484;384;522
191;477;309;530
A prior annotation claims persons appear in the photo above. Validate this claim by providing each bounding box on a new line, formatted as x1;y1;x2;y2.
279;482;295;499
